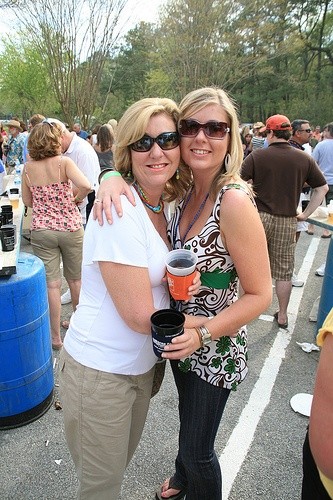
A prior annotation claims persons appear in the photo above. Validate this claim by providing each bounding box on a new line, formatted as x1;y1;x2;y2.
92;87;275;500
299;307;333;500
240;114;329;329
0;113;333;289
21;120;92;350
59;96;180;500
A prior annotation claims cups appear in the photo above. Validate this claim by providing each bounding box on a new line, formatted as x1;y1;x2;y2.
0;228;15;252
149;308;186;357
8;194;20;208
165;249;199;301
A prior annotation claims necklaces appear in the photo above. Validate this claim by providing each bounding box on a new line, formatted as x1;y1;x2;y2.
133;181;164;214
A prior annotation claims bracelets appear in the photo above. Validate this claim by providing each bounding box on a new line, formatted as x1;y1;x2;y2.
100;170;121;184
75;196;80;201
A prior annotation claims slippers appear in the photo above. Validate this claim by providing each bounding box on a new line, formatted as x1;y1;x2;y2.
51;342;61;350
320;232;331;238
156;474;185;500
305;228;313;235
274;309;288;329
62;320;70;329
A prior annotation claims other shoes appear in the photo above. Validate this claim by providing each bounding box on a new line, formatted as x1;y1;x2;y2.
291;275;305;287
315;262;325;276
60;289;72;305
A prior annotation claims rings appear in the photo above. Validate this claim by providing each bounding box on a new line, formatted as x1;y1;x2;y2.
94;199;101;205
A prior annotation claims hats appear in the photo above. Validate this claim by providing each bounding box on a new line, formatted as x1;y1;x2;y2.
254;122;264;129
5;120;23;131
259;114;289;133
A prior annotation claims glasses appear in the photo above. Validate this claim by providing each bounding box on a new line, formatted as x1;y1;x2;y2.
299;128;312;133
179;119;230;139
131;132;180;153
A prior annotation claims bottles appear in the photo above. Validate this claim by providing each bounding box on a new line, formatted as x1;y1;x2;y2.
13;160;22;197
0;204;14;225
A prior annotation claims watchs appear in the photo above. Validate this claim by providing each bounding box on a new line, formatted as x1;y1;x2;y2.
194;325;212;348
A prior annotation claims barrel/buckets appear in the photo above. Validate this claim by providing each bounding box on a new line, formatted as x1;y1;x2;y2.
0;251;56;429
